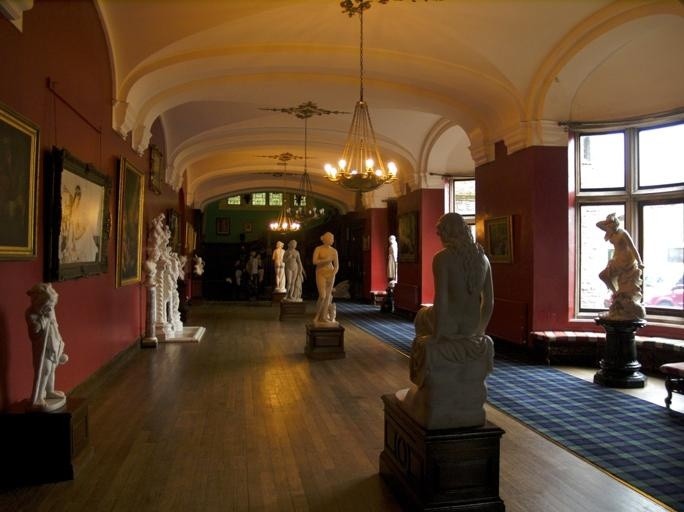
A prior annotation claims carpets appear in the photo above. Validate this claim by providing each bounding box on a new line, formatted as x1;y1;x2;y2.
331;300;684;512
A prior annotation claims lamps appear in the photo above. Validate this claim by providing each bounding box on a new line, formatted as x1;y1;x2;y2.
269;108;325;234
322;1;399;192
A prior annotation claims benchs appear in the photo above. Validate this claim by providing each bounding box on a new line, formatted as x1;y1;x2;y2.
527;325;684;406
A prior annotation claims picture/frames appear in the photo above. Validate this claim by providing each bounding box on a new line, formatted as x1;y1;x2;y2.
166;207;182;254
116;155;145;290
395;209;420;265
483;213;515;265
149;144;164;194
0;100;40;260
44;145;112;283
215;217;231;236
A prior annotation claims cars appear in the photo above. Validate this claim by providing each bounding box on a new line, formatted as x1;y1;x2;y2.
650;284;684;306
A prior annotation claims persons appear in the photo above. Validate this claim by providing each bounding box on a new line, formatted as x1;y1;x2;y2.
414;211;494;341
312;232;339;325
386;234;399;281
27;281;68;407
64;185;98;260
142;214;205;287
247;250;264;282
597;213;647;321
270;239;307;299
